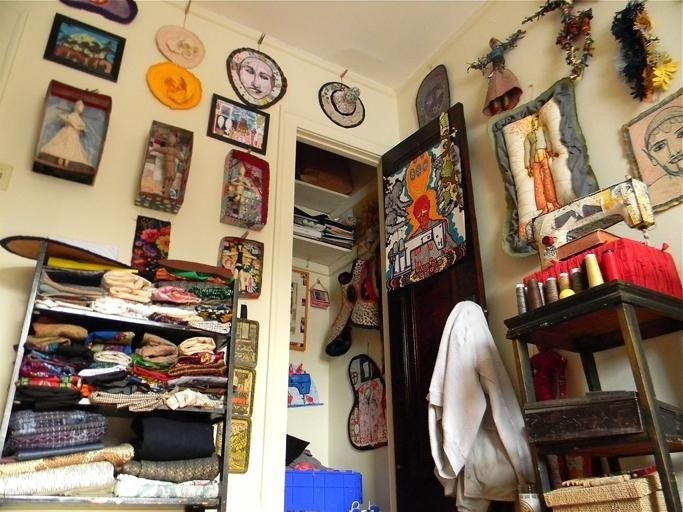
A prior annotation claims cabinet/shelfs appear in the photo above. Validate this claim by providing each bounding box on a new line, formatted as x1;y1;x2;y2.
0;241;240;512
292;142;353;268
502;279;682;512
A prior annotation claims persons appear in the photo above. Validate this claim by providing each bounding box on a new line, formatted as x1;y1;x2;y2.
44;98;90;165
640;105;683;189
236;56;275;100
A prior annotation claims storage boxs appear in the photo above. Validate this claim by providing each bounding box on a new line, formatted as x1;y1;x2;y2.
283;469;362;512
541;471;668;512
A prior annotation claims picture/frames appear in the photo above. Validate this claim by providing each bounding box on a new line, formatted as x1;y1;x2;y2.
289;268;308;352
206;93;270;155
42;11;127;84
215;318;259;475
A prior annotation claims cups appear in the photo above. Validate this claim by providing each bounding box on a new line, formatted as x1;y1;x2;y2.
517;482;543;512
